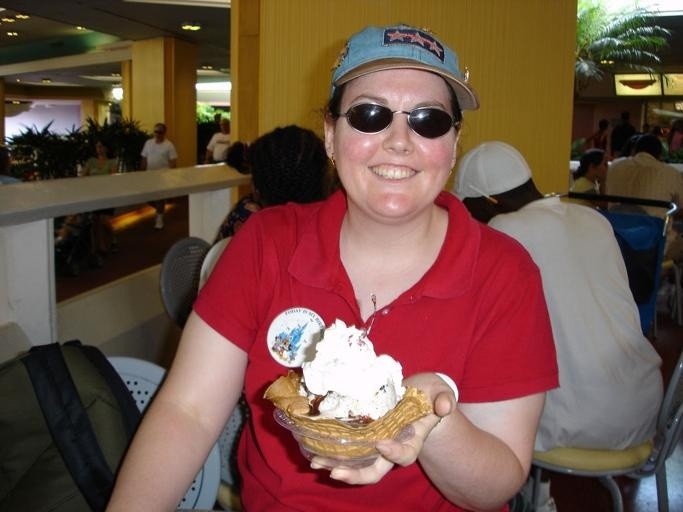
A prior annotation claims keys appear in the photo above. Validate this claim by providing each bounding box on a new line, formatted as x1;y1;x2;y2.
203;118;230;164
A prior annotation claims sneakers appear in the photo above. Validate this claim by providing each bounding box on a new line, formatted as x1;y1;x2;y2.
156;214;165;221
154;221;163;228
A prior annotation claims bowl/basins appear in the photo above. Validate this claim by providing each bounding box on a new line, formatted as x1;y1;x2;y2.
273;408;415;468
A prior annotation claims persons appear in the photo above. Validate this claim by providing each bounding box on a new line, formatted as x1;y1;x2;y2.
0;146;22;185
605;133;683;326
106;23;560;512
140;123;178;229
667;119;682;158
197;123;338;296
80;137;120;249
593;118;609;155
610;111;637;157
568;149;608;210
449;141;664;511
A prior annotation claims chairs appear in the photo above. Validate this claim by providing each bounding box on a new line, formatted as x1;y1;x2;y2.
653;260;681;340
217;393;249;512
107;356;221;512
159;237;212;330
531;352;683;512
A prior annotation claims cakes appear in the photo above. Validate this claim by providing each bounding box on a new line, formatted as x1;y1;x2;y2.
293;291;407;424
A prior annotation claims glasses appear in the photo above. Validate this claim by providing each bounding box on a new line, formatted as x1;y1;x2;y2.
338;102;458;139
153;130;162;134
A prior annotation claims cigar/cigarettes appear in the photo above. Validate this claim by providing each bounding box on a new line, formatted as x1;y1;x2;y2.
467;184;497;203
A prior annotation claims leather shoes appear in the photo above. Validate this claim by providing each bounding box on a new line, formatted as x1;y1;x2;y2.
95;253;104;265
113;242;120;252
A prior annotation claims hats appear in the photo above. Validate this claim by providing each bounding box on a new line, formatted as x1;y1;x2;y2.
332;23;479;111
449;141;531;201
